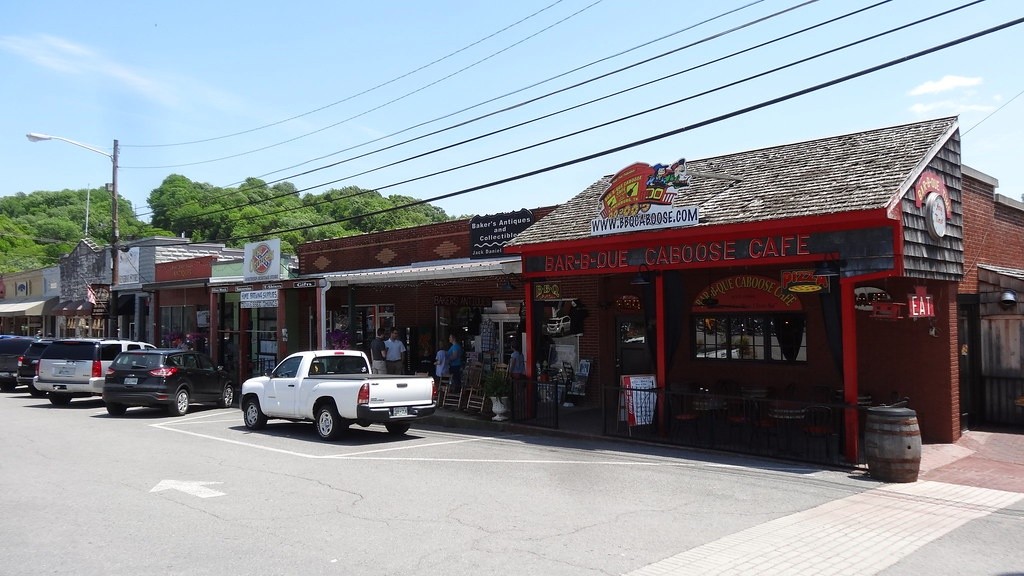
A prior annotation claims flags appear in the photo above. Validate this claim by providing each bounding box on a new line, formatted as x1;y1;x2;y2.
87;288;96;305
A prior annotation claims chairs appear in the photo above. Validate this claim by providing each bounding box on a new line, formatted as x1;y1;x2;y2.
437;361;509;412
669;377;836;459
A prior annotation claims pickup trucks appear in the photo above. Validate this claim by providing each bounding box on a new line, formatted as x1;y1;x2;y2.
623;337;740;359
241;349;437;443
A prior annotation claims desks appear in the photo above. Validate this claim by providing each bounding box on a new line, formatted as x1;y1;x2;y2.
767;401;807;458
692;397;729;448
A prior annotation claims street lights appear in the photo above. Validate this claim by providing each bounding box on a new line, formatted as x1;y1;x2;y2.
26;132;120;338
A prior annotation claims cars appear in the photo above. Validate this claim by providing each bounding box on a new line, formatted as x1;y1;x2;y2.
103;348;234;418
0;335;33;392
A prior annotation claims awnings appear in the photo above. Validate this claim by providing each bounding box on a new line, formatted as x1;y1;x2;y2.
61;300;85;316
77;302;94;315
0;296;59;317
50;301;73;316
116;294;136;315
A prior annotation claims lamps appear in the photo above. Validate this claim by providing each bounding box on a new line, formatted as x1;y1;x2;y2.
598;279;613;310
701;275;718;306
630;263;661;285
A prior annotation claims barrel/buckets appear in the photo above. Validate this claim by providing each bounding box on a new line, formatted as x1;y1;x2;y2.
864;406;922;483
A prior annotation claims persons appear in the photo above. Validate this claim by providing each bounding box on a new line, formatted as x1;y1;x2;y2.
420;351;436;374
36;330;42;336
446;333;464;394
509;340;525;378
370;328;387;374
383;327;406;374
184;335;194;350
434;340;450;381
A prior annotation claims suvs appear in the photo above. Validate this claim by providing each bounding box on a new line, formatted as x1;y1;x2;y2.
18;337;57;398
32;337;157;406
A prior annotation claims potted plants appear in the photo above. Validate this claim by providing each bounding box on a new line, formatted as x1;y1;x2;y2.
482;370;514;422
540;371;549;382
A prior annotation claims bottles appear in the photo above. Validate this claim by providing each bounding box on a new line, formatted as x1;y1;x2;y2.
542;360;548;373
536;361;541;375
